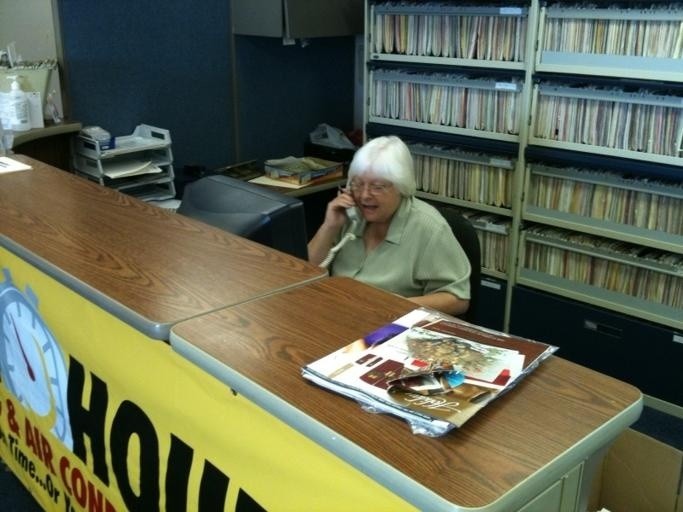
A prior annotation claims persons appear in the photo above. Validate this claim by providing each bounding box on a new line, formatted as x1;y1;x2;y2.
307;134;472;316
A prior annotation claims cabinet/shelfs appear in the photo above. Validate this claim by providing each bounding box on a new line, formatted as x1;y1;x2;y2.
363;0;536;331
70;118;176;202
232;0;364;40
509;0;683;419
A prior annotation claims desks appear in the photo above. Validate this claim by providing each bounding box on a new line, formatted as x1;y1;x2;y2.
246;167;350;198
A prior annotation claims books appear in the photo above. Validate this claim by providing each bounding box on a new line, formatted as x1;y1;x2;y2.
521;226;682;308
372;12;528;62
535;86;682;158
462;210;508;274
369;68;525;135
542;19;681;60
300;305;561;436
404;138;513;208
529;166;681;235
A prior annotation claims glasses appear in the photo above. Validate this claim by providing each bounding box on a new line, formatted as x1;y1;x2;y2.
351;180;394;194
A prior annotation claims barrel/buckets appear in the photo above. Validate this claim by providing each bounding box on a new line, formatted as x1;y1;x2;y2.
6;74;31;132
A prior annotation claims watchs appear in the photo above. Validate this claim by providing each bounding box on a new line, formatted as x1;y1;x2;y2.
0;266;78;454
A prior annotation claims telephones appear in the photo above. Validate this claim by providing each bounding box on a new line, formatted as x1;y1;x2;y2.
344;177;363;222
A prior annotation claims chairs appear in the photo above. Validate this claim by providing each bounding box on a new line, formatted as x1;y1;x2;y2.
433;206;482;324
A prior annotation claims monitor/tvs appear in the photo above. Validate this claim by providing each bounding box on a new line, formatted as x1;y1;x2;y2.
177;175;309;261
